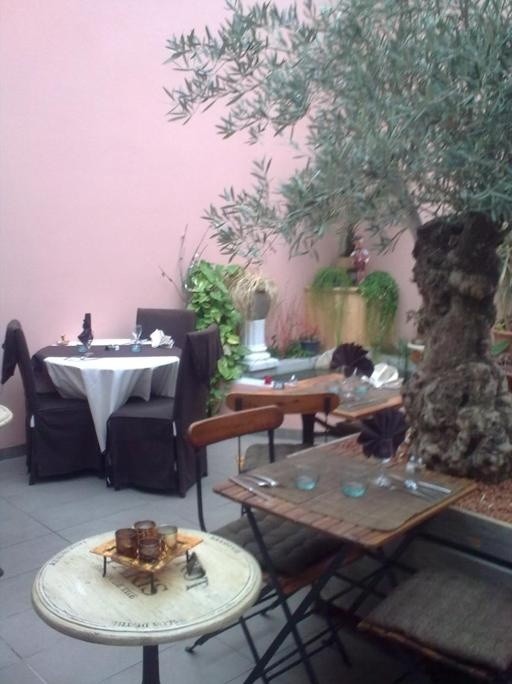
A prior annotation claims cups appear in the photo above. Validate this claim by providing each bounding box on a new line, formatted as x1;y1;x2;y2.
115;528;137;555
403;453;427;490
131;325;142;351
135;520;156;540
139;540;159;560
340;466;366;497
157;524;177;550
296;465;318;489
371;438;393;487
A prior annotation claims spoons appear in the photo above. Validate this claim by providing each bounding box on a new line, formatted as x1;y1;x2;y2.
243;475;270;487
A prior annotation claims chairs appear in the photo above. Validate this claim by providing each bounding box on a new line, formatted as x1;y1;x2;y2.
182;404;355;654
357;562;512;684
132;308;199;350
224;390;341;519
107;324;224;498
0;319;106;485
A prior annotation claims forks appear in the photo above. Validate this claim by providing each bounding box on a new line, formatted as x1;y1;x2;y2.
248;472;281;487
